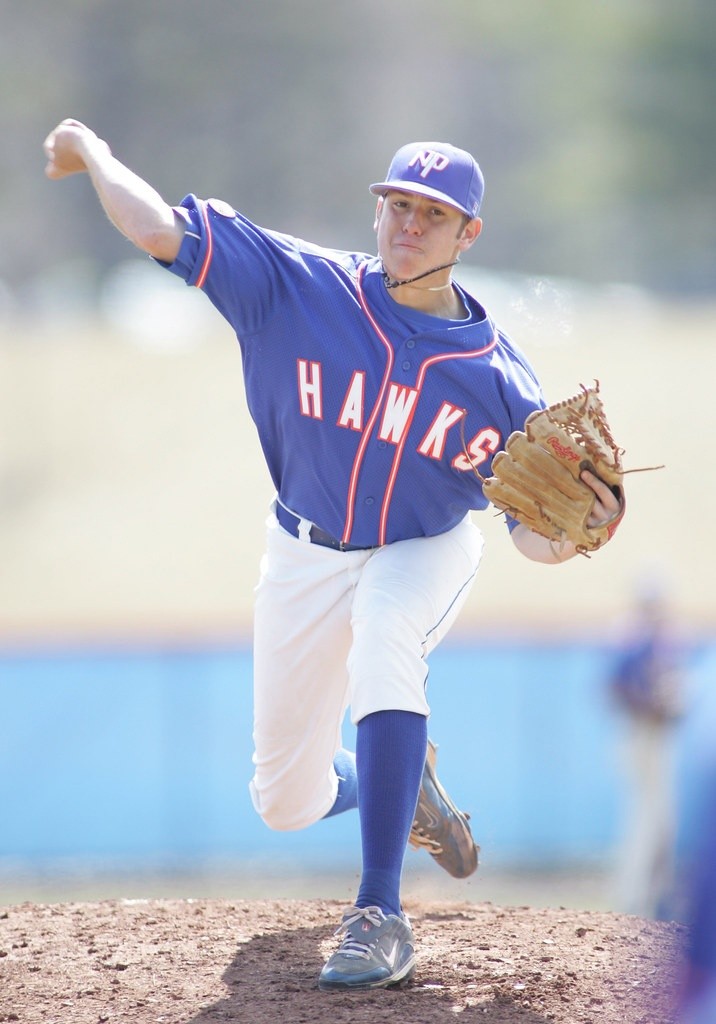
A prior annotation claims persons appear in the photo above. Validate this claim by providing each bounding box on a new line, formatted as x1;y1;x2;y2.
45;119;623;994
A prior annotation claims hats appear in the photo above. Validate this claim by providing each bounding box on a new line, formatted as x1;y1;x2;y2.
368;141;485;221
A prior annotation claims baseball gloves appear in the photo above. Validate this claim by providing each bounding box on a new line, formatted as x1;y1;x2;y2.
480;385;628;556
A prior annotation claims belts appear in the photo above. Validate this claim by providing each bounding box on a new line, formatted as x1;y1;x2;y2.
274;500;380;553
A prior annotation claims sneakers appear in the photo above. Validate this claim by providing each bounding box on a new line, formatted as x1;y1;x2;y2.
318;904;419;990
408;737;482;879
